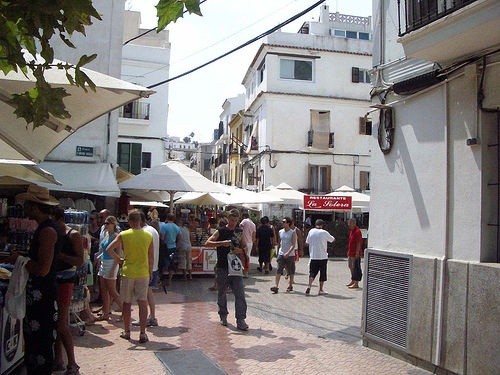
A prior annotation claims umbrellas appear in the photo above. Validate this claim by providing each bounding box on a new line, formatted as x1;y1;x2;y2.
0;50;369;222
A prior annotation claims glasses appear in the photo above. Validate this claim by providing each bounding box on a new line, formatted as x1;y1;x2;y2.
103;222;109;225
282;221;286;223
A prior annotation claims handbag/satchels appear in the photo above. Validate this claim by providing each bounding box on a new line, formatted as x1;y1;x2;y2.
5;255;32;319
227;247;249;277
351;257;362;281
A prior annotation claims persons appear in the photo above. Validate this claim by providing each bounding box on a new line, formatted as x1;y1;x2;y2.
346;218;363;288
6;184;63;375
44;208;83;375
85;206;313;343
304;218;336;296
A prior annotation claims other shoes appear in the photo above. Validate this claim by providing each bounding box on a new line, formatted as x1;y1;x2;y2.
237;323;249;330
148;315;158;325
286;287;293;291
132;319;151;326
257;267;262;272
54;361;66;370
220;319;227;326
140;334;148;343
346;282;358;288
65;365;80;375
306;288;310;296
271;287;279;293
95;315;110;320
319;291;328;295
120;331;130;339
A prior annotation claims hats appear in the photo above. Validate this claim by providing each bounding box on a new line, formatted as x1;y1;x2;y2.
315;219;326;224
16;185;60;205
